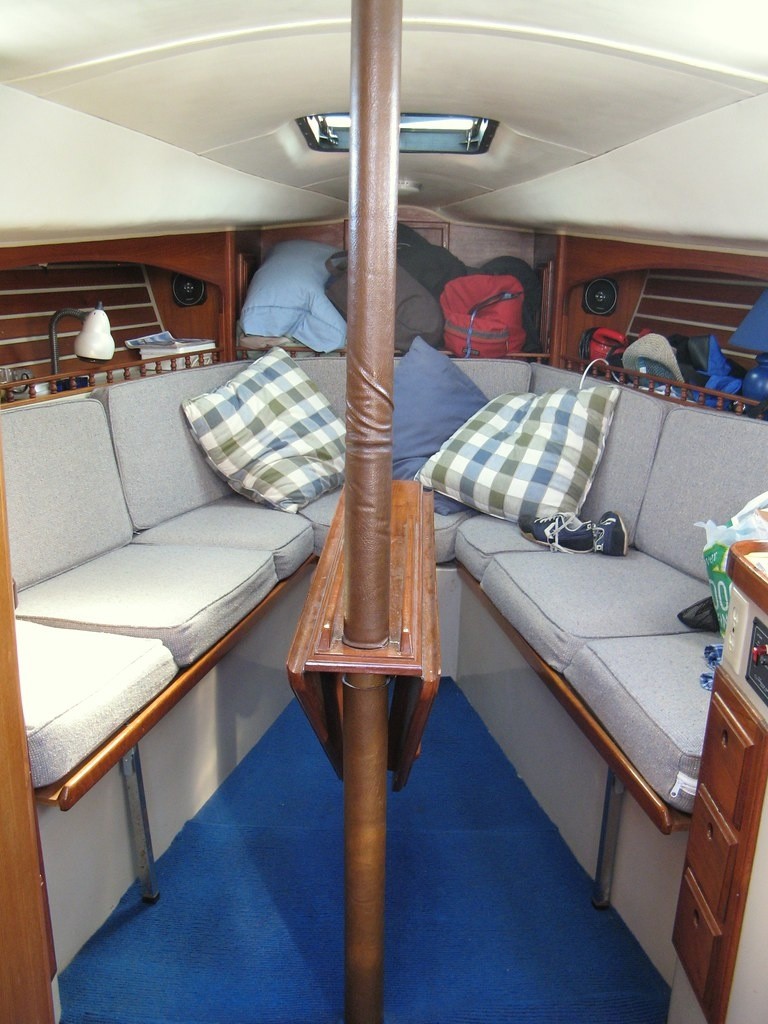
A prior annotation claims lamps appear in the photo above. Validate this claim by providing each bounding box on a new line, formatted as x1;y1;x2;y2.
50;301;115;374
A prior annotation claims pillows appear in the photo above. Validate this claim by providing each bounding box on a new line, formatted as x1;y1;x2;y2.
391;337;489;517
412;386;621;523
182;347;346;513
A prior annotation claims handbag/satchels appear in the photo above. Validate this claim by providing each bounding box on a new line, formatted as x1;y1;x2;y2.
325;250;444;353
669;333;748;410
439;273;526;360
694;489;768;639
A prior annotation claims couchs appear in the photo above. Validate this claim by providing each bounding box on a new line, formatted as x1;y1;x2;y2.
0;352;768;990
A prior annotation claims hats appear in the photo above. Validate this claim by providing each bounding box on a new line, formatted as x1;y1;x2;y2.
621;332;685;388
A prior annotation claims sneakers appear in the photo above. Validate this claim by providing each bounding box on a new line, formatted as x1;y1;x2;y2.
591;511;628;556
517;511;594;555
